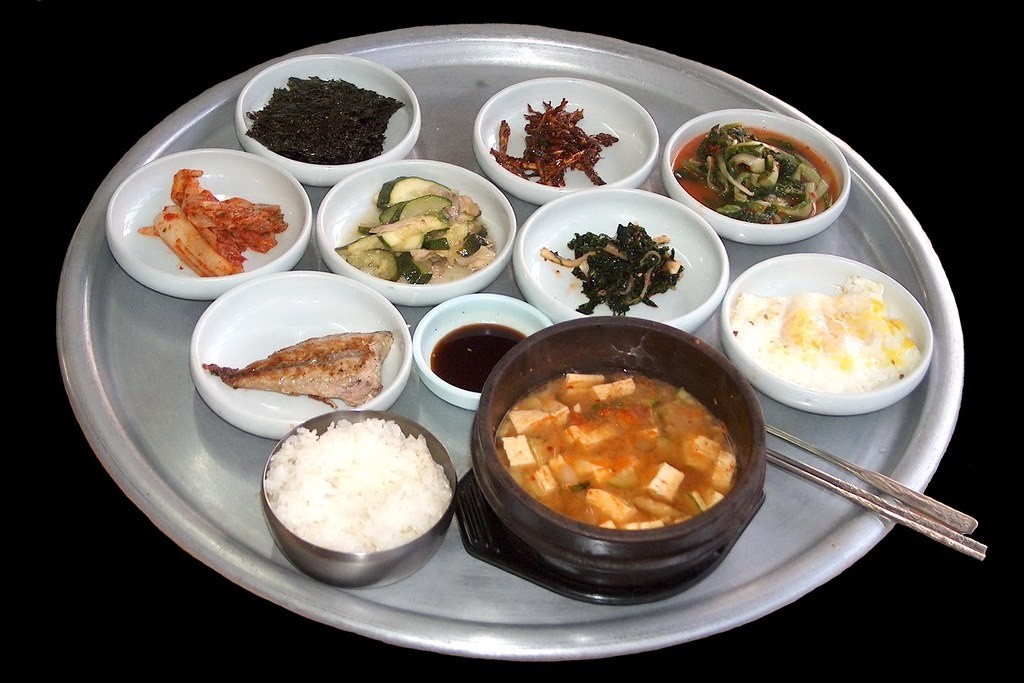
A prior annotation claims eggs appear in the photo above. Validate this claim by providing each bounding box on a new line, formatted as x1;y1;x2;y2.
730;276;922;394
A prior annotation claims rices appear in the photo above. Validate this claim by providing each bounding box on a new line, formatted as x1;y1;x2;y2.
266;417;452;554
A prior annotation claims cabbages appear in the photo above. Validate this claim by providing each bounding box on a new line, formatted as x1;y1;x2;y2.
137;169;290;278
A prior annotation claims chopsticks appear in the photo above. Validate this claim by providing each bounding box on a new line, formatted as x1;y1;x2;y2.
763;422;988;561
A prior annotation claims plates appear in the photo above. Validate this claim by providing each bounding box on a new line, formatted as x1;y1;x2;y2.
719;251;933;416
413;293;552;413
189;269;413;441
316;159;517;306
105;146;312;301
234;54;420;189
512;189;729;333
474;77;658;205
662;109;851;244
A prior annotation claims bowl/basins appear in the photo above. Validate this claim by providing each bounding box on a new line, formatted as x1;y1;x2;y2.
470;315;766;586
262;410;459;590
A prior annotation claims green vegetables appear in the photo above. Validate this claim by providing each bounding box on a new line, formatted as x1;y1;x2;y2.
568;397;626;492
567;222;684;316
673;122;832;224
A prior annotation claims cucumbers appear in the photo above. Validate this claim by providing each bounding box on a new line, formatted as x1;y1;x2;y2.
335;176;488;284
